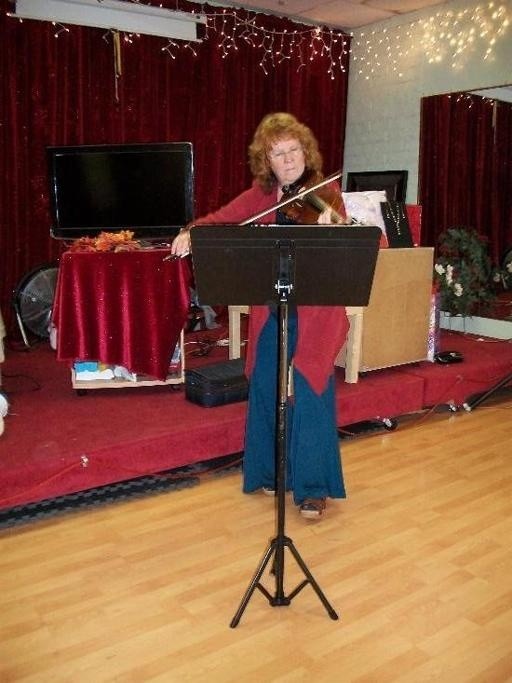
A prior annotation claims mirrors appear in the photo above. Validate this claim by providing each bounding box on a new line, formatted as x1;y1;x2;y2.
418;83;511;322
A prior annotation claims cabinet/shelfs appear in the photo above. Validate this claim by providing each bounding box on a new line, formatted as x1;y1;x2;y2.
61;249;186;396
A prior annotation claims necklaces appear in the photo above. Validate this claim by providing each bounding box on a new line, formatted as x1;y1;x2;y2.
386;202;407;236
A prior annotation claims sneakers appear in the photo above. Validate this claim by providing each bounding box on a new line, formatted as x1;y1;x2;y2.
260;487;276;496
298;498;328;519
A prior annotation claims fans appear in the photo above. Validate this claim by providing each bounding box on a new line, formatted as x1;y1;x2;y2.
10;261;58;350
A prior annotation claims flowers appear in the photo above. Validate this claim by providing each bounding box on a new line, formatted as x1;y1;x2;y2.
434;225;512;317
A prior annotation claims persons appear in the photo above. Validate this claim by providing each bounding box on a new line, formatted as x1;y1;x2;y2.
171;113;351;518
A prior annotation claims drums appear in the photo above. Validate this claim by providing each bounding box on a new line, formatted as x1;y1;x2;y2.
12;261;59;339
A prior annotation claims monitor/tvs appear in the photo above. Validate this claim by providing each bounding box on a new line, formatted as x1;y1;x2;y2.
346;170;408;205
45;142;195;242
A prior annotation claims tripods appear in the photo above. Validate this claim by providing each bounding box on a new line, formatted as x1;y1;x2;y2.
230;294;339;629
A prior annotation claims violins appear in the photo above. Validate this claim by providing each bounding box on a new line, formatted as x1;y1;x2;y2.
279;165;364;226
